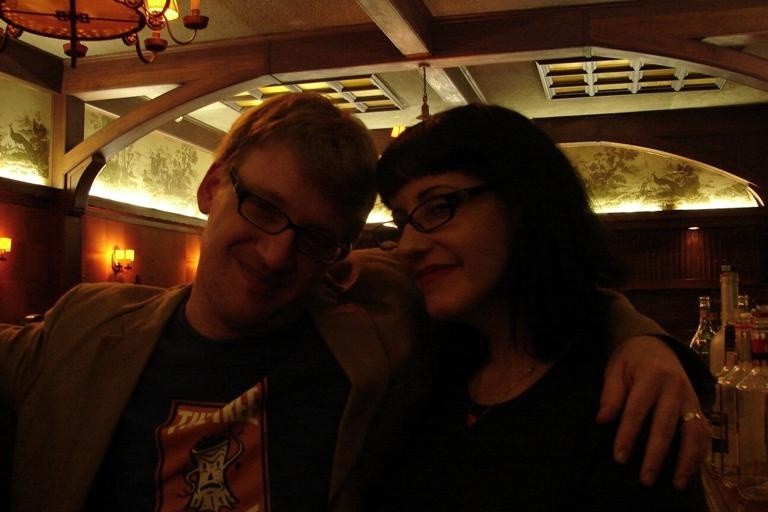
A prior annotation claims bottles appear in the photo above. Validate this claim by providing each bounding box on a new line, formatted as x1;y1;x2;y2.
688;268;768;504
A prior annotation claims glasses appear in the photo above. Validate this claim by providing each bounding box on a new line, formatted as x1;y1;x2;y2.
227;169;346;267
373;182;497;252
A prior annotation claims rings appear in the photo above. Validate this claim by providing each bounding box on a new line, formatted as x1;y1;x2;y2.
682;411;700;422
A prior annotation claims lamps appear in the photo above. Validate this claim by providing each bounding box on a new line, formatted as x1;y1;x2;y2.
112;246;135;274
0;0;209;69
0;237;12;259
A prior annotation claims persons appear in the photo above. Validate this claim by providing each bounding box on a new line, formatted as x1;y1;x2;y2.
0;90;718;511
357;96;713;508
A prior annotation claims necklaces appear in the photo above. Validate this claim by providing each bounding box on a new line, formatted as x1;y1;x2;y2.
490;365;538;410
